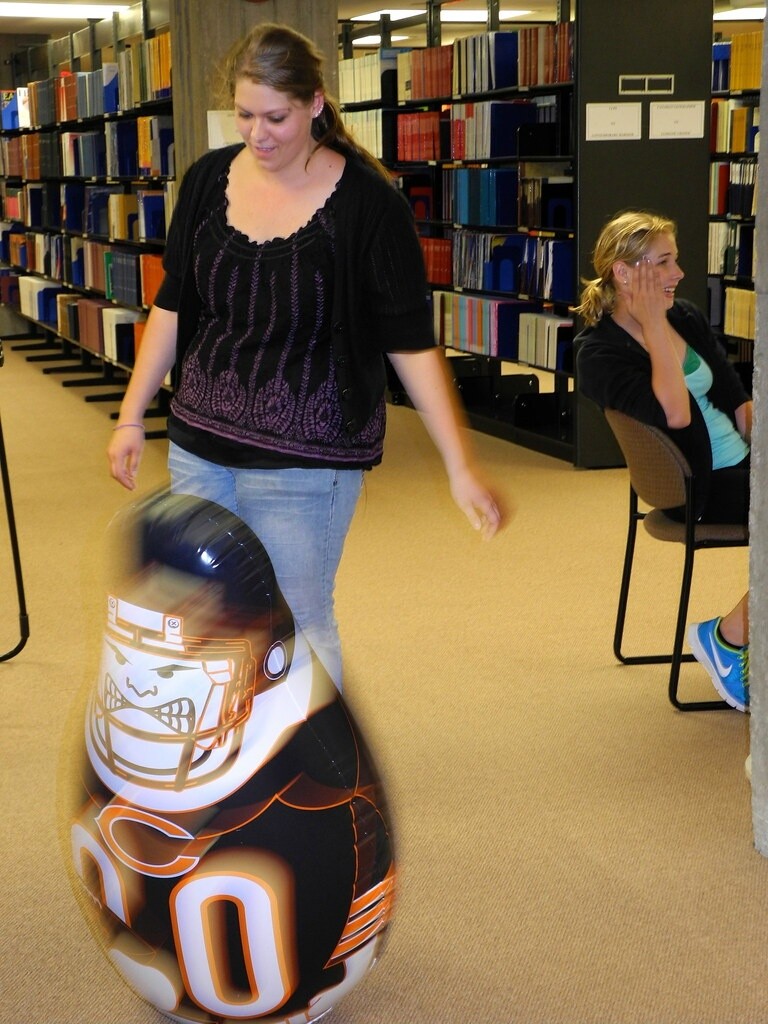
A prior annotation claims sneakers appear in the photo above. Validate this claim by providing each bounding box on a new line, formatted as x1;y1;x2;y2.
688;617;750;714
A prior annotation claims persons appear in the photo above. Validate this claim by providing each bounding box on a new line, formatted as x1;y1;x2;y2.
569;211;752;714
108;22;502;695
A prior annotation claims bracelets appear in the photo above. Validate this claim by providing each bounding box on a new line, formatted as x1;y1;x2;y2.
113;423;146;431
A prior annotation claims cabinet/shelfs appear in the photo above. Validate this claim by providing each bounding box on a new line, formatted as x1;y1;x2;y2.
0;0;767;469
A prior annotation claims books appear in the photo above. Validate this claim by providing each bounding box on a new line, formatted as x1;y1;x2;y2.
338;22;574;371
0;32;176;387
706;31;765;363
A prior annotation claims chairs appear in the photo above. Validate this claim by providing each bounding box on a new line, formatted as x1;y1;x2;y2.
605;407;749;713
